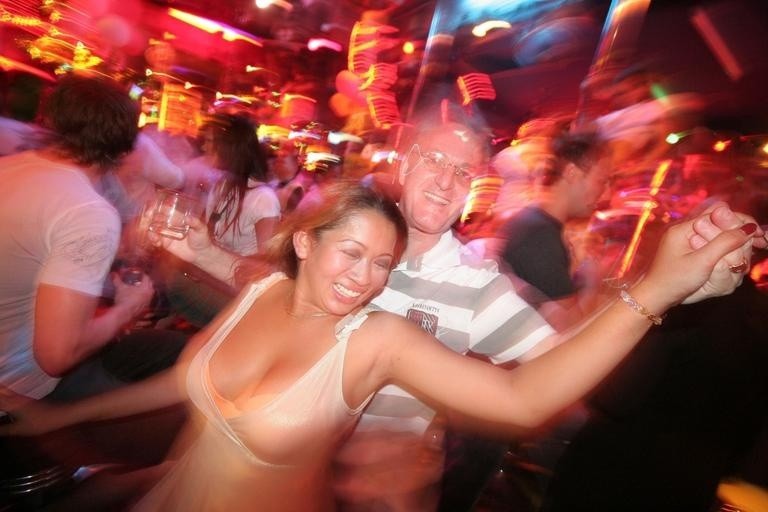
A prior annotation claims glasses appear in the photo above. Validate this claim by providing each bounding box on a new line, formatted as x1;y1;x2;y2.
412;147;477;186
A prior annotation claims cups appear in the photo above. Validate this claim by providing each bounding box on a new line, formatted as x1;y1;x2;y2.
148;187;200;241
